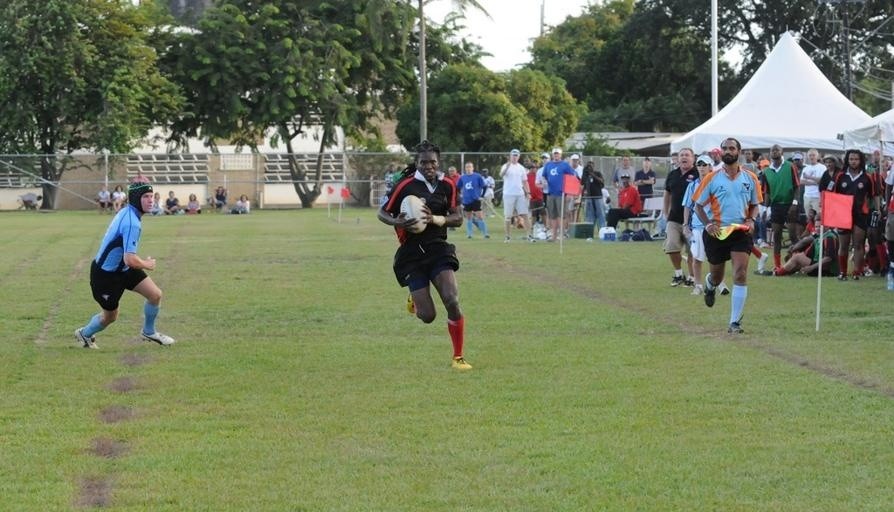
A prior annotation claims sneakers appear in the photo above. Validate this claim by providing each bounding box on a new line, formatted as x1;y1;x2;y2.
758;253;768;272
669;276;684;286
702;284;716;307
406;291;417;315
719;284;729;295
727;322;744;333
503;237;511;241
852;272;860;279
74;328;101;348
483;235;490;239
690;285;700;295
683;278;694;287
467;236;471;240
527;235;537;240
140;330;174;345
838;274;847;281
449;357;471;368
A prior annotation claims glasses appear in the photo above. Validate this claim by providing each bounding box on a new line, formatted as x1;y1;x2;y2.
695;163;706;165
532;160;538;162
542;157;548;158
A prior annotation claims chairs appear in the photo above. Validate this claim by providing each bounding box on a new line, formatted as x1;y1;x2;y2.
621;198;664;235
766;229;785;245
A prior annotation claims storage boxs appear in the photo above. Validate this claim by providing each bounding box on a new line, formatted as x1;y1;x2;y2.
568;222;594;240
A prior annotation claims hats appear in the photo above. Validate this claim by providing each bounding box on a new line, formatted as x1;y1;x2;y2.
551;148;562;153
510;149;520;154
694;155;714;166
791;154;802;159
570;154;580;159
540;153;551;158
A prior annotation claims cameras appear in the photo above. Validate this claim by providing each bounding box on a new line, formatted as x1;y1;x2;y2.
582;165;594;184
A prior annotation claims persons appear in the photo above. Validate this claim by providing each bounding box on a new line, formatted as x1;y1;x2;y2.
683;155;730;296
76;175;176;348
565;155;584;239
499;149;536;244
481;169;495;217
111;184;127;213
865;153;894;291
457;162;489;240
19;193;43;210
185;194;201;214
772;215;840;276
790;150;807;244
742;149;772;179
663;148;699;287
379;141;474;371
694;137;763;334
634;158;656;197
228;194;249;215
583;158;607;231
96;184;112;210
151;192;163;217
819;154;838;206
543;149;577;241
385;164;408;195
838;150;877;282
521;152;549;233
163;190;178;215
800;208;818;239
607;173;641;229
801;148;827;216
448;165;460;189
209;186;228;211
764;145;800;269
614;155;637;185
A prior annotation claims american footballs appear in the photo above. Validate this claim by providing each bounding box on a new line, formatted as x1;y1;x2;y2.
402;196;428;232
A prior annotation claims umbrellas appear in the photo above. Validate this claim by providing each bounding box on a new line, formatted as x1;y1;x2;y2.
669;31;876;155
837;107;894;147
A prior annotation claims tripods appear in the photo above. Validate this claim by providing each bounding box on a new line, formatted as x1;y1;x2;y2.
576;182;601;224
